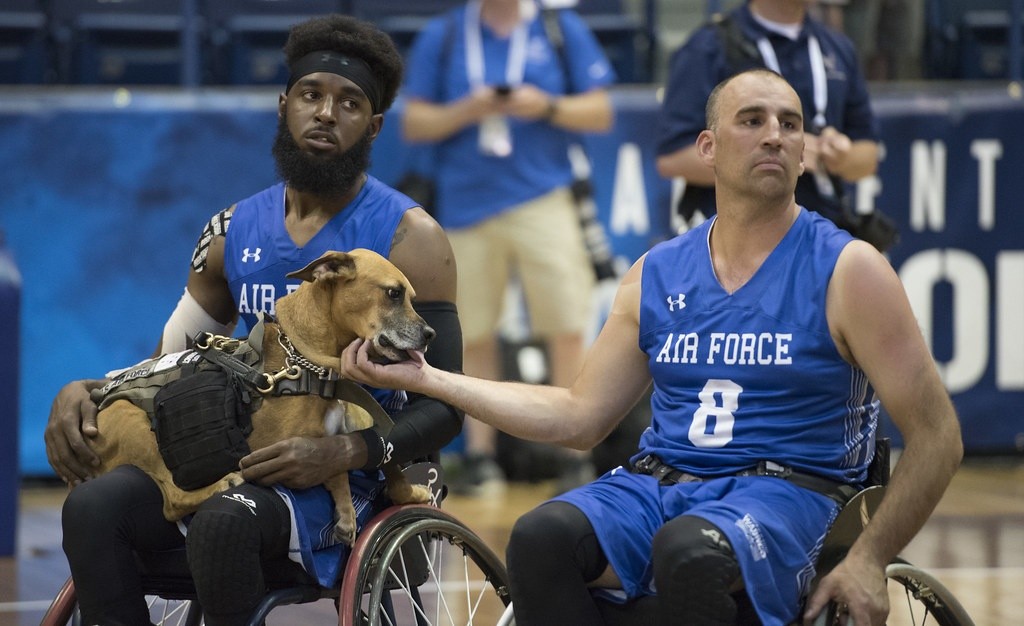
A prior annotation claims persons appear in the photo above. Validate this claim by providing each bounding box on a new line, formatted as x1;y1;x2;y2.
339;0;963;626
44;13;464;626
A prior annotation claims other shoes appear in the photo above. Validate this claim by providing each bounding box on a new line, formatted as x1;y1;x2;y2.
460;454;507;498
554;458;597;499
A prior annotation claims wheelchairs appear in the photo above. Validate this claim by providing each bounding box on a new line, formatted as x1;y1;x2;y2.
496;426;976;626
36;453;519;626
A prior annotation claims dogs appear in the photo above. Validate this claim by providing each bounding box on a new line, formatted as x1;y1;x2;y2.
68;248;440;548
